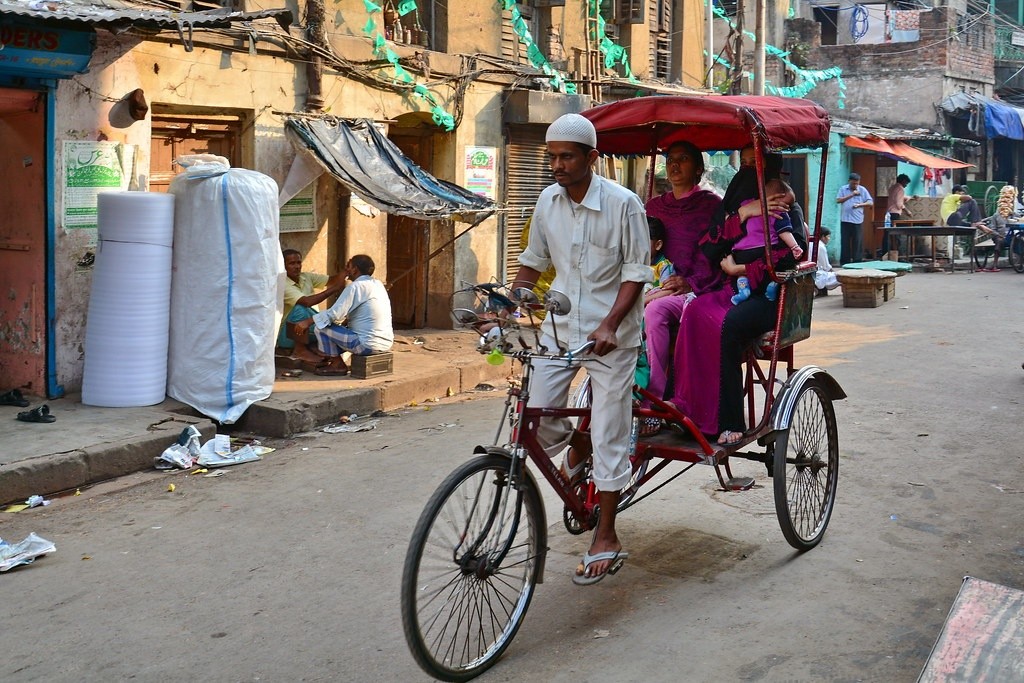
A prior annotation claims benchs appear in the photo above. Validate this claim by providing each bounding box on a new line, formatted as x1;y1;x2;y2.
739;259;816;361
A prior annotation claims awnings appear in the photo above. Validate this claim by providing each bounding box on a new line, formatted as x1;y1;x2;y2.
0;0;293;49
844;135;976;169
272;108;501;290
944;91;1024;140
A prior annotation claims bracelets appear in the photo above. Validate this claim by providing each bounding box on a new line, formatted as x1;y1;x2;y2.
791;244;799;250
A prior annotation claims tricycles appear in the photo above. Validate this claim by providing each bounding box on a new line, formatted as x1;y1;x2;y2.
973;206;1024;275
397;91;852;683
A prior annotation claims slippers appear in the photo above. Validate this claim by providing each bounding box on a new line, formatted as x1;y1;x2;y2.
572;547;618;585
315;364;347;376
560;446;590;484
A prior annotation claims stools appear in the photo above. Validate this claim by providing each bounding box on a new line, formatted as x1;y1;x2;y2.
349;352;393;378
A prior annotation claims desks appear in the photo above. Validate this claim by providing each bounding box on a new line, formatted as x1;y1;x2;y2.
872;219;977;273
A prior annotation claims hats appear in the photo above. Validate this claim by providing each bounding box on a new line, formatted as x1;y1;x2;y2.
546;113;597;148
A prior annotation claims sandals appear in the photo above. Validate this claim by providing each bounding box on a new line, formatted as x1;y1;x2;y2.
717;430;743;446
671;423;688;437
638;417;661;437
651;401;679;413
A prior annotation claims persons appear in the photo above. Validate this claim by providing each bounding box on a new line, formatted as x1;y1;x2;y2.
836;173;873;268
882;174;919;262
808;226;841;290
278;249;394;375
941;184;992;233
474;108;654;586
632;141;805;445
